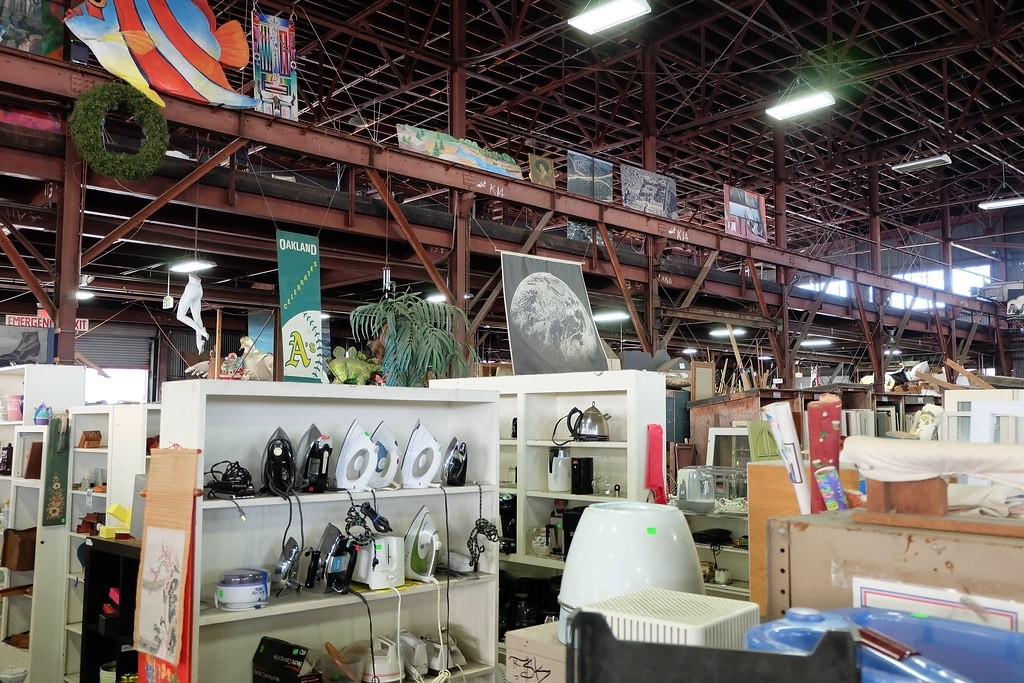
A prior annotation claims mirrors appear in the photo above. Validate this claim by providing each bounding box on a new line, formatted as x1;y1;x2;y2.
690;361;715;401
704;427;751;494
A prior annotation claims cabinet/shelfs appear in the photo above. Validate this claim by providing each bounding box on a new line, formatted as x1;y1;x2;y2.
0;364;1024;683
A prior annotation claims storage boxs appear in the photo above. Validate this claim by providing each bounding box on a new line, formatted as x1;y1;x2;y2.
505;620;579;683
254;634;322;683
584;587;760;651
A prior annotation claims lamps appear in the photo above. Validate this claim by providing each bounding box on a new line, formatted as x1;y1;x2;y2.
979;161;1024;210
163;144;218;309
892;138;954;173
568;0;652;36
424;262;474;303
766;75;835;120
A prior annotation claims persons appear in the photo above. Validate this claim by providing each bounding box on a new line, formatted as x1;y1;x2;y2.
74;350;111;378
177;273;209;355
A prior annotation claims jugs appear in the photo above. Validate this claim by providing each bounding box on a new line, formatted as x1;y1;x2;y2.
532;526;552;556
546;446;571;492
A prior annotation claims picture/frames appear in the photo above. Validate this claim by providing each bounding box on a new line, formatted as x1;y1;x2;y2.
529;153;556;189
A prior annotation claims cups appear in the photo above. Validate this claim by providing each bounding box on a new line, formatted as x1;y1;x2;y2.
570;457;594;495
321;652;364;683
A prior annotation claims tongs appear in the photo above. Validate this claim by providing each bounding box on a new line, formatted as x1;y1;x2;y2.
324;641;355;681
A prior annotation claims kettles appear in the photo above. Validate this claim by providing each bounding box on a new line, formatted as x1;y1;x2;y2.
567;401;612;441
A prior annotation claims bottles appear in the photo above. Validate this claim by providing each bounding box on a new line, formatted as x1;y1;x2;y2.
506;465;515;485
1;499;10;532
82;468;91;491
0;443;13;475
